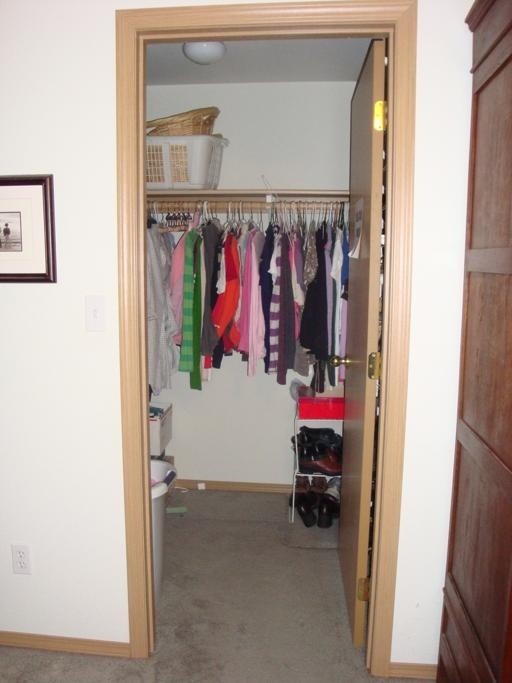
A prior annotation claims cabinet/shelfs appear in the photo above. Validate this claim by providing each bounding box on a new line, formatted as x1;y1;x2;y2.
289;399;342;526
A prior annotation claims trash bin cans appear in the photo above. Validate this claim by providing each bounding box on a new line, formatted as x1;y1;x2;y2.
150;459;177;603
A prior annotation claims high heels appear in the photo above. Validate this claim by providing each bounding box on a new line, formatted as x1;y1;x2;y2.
289;425;349;529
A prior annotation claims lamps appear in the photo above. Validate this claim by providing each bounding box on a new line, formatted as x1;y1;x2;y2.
183;41;225;65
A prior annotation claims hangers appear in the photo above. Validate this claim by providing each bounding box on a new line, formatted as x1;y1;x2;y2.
146;201;348;233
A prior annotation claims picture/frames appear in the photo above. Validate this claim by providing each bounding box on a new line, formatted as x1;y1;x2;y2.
0;171;59;283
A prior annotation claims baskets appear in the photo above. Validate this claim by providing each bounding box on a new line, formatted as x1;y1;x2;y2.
145;105;229;192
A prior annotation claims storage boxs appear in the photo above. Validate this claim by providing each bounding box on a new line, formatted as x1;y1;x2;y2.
295;384;345;419
150;401;175;456
